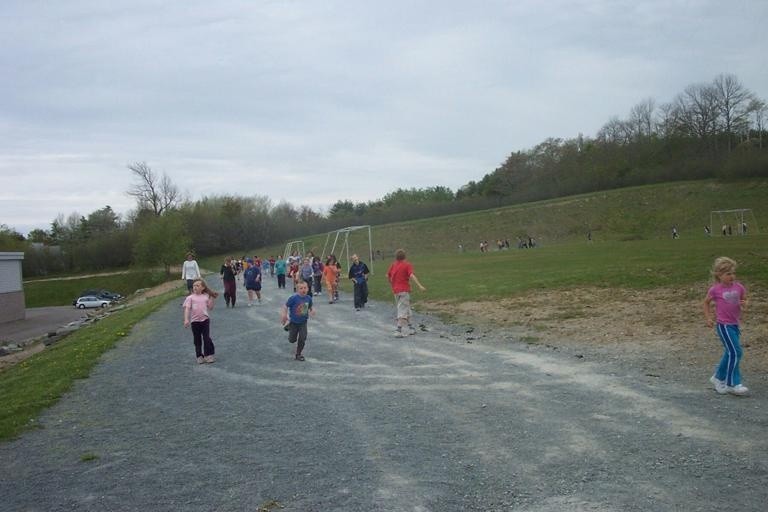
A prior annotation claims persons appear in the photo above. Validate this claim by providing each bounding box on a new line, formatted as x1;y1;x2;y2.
349;253;370;311
281;280;316;361
672;225;680;240
477;235;538;253
182;278;218;362
386;248;426;338
703;256;751;399
220;251;342;308
704;221;747;237
182;252;200;294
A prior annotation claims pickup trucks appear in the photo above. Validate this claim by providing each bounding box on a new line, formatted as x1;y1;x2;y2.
72;289;121;305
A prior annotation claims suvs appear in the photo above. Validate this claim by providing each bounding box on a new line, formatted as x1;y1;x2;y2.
75;295;113;309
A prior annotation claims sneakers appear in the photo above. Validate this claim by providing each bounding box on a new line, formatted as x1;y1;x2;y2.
295;353;305;361
394;331;403;338
409;328;418;335
284;323;290;331
709;375;726;394
197;355;213;364
725;383;749;396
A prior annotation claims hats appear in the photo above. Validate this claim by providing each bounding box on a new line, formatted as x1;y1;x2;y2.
244;258;253;263
